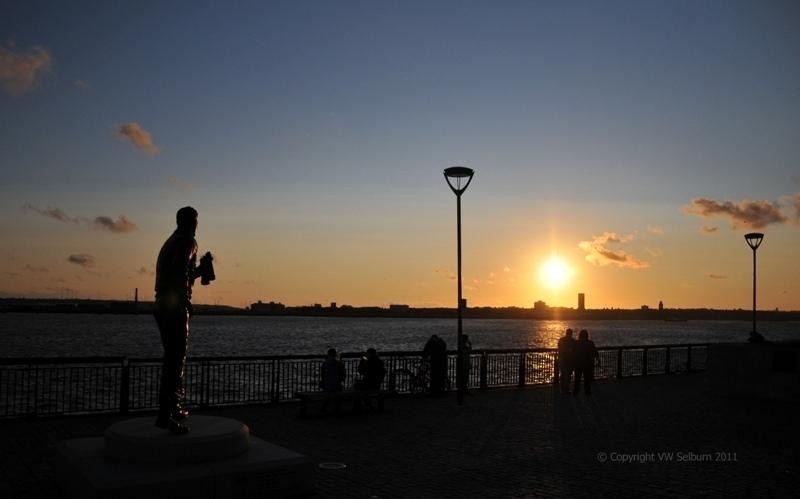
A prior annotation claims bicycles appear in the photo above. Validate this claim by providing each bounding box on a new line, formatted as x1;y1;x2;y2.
389;356;430;394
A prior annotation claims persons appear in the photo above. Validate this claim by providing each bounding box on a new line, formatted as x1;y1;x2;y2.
421;333;450;397
151;203;214;433
351;348;386;391
573;330;599;398
556;327;577;397
318;347;347;394
460;333;472;392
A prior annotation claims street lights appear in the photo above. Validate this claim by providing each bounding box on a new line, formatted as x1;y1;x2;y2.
443;166;474;406
744;233;764;334
